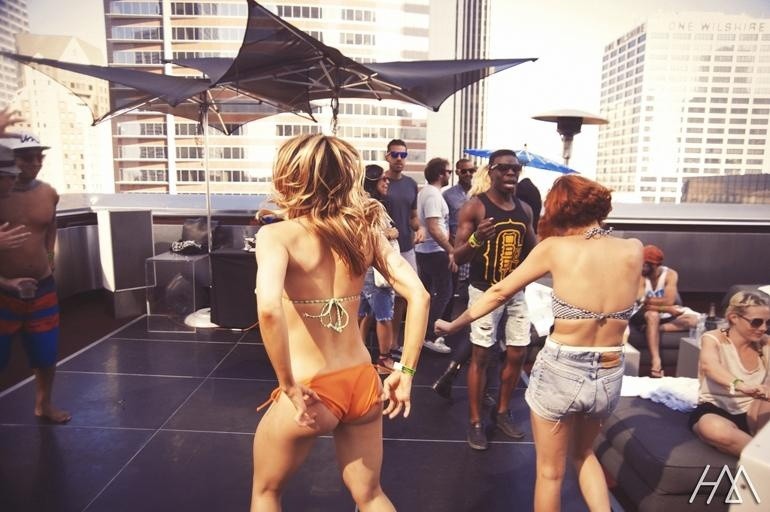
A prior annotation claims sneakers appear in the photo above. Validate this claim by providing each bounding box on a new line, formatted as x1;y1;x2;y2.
467;420;488;450
423;338;451;353
390;347;402;359
490;407;526;438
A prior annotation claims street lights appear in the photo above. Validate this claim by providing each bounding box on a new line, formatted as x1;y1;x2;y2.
529;114;612;177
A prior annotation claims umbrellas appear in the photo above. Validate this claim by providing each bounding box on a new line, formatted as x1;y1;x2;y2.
161;2;538;137
463;142;582;176
1;51;320;252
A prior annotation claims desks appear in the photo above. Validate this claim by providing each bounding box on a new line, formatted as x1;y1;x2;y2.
144;247;209;334
676;335;702;378
207;246;257;329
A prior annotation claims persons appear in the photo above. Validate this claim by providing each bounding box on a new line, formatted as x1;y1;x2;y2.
0;142;37;301
248;132;432;511
433;173;645;512
1;131;72;424
359;139;770;458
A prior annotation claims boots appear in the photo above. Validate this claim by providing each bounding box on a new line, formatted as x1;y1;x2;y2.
432;356;462;398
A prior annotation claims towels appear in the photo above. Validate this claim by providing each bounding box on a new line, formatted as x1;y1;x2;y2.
621;374;703;415
524;279;556;345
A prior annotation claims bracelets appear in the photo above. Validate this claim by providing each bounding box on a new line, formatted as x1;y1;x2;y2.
392;360;416;378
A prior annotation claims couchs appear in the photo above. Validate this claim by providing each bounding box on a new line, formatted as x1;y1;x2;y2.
592;374;742;512
526;323;703;377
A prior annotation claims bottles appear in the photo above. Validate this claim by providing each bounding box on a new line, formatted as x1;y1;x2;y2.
704;301;717;330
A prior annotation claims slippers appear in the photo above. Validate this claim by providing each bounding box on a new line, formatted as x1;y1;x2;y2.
650;368;664;378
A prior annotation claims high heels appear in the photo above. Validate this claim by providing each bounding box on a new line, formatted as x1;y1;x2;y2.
376;353;398;375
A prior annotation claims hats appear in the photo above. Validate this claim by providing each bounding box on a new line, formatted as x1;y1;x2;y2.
0;145;23;174
0;132;50;150
644;245;664;264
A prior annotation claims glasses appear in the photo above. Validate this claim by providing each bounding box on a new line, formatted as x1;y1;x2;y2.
490;163;522;171
15;153;45;161
389;152;407;158
737;314;770;328
444;170;451;174
459;169;476;174
380;177;387;182
